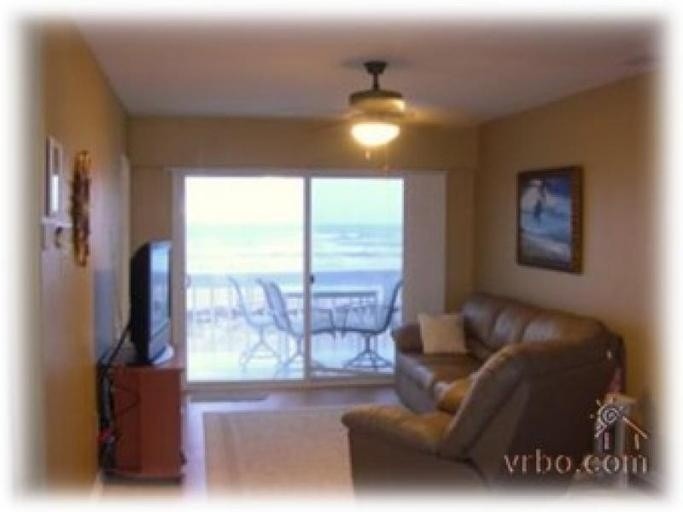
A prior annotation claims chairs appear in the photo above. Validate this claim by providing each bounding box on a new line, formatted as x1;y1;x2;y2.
227;273;402;376
340;339;617;496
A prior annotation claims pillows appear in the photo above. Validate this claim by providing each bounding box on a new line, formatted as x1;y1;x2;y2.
419;312;466;356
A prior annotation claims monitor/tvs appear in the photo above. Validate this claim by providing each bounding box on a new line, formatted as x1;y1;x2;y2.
131;240;174;366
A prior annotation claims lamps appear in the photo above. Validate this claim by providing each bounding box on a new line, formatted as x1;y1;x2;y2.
334;60;410;148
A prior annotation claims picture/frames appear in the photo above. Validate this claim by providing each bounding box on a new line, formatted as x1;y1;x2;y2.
513;165;583;274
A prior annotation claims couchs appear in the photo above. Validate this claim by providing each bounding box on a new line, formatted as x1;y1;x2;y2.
392;290;622;419
